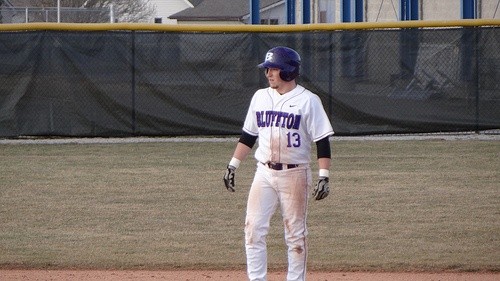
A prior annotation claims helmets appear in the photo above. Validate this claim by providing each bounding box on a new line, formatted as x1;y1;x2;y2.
256;47;302;81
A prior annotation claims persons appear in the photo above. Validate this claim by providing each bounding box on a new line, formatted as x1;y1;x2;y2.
223;46;335;281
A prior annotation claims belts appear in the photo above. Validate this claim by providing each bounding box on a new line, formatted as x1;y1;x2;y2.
260;162;298;170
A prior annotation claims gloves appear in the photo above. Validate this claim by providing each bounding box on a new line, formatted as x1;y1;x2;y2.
223;157;241;192
311;169;329;200
